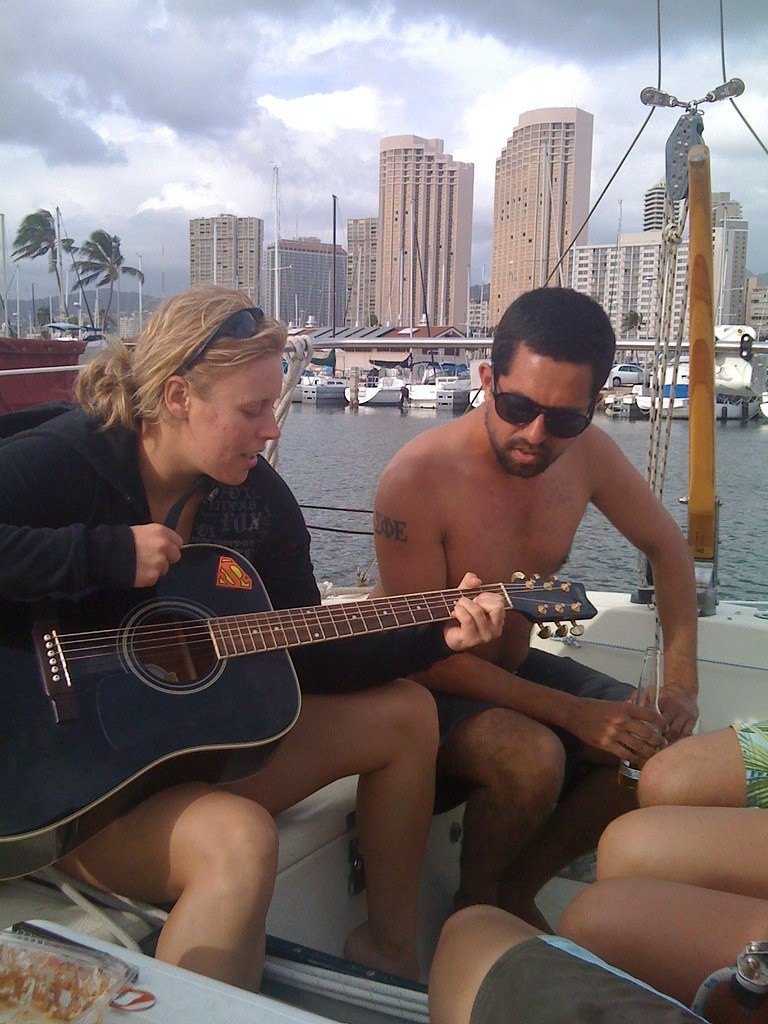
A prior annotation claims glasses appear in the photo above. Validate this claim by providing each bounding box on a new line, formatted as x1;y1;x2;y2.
491;363;595;438
175;308;264;377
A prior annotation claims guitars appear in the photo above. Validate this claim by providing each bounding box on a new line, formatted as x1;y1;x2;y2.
2;541;599;885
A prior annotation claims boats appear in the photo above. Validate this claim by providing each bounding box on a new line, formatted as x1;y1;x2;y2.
251;144;768;421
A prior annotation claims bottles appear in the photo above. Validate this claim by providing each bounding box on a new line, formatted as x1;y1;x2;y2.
613;647;663;793
706;940;768;1024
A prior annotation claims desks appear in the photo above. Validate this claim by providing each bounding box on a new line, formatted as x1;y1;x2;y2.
1;920;343;1024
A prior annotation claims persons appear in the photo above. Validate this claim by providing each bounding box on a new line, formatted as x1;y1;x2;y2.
0;284;506;996
364;286;701;937
426;709;768;1024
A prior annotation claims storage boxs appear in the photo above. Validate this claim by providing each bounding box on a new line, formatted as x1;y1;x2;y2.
263;774;468;986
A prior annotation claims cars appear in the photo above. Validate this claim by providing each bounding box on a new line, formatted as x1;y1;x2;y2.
607;364;652;389
